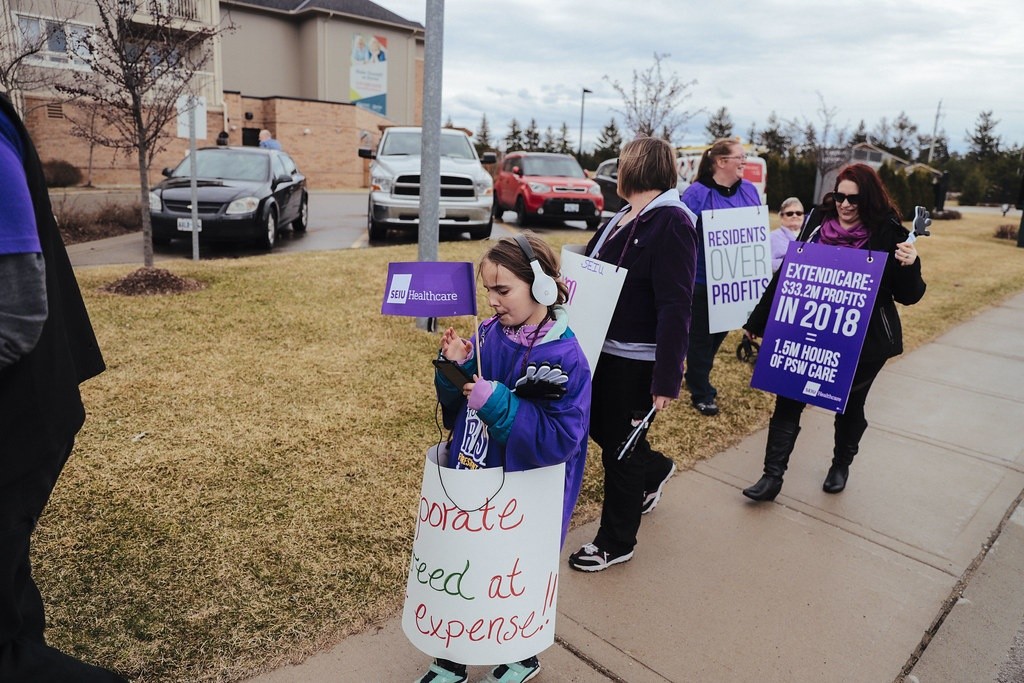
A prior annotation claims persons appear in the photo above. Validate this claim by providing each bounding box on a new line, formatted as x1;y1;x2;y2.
415;232;593;683
570;137;700;574
740;163;927;502
1015;174;1024;247
258;129;280;149
682;137;762;415
0;83;132;683
216;131;229;145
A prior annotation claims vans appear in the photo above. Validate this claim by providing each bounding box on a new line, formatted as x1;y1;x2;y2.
672;155;768;207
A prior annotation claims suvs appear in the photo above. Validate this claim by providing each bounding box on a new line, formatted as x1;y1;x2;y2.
592;157;629;213
148;146;311;252
356;125;499;245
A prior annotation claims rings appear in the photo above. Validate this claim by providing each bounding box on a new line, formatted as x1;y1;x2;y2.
904;253;910;258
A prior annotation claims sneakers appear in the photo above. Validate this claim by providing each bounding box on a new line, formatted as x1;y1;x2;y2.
569;542;634;573
690;385;718;415
642;459;676;514
413;658;468;683
478;655;541;683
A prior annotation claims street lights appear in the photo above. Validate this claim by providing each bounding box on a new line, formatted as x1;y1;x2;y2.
578;87;594;159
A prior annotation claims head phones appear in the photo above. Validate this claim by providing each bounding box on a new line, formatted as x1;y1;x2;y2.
512;233;558;305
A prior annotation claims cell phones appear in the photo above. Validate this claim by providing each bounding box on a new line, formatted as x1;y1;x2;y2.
431;359;474;391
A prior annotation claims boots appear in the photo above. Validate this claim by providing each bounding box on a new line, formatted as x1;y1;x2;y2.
743;418;801;502
823;413;868;494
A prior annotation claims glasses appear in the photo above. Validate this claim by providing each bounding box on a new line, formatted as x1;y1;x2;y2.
782;211;805;217
721;154;748;161
833;192;859;204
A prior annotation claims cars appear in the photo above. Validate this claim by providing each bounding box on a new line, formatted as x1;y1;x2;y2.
493;151;604;229
